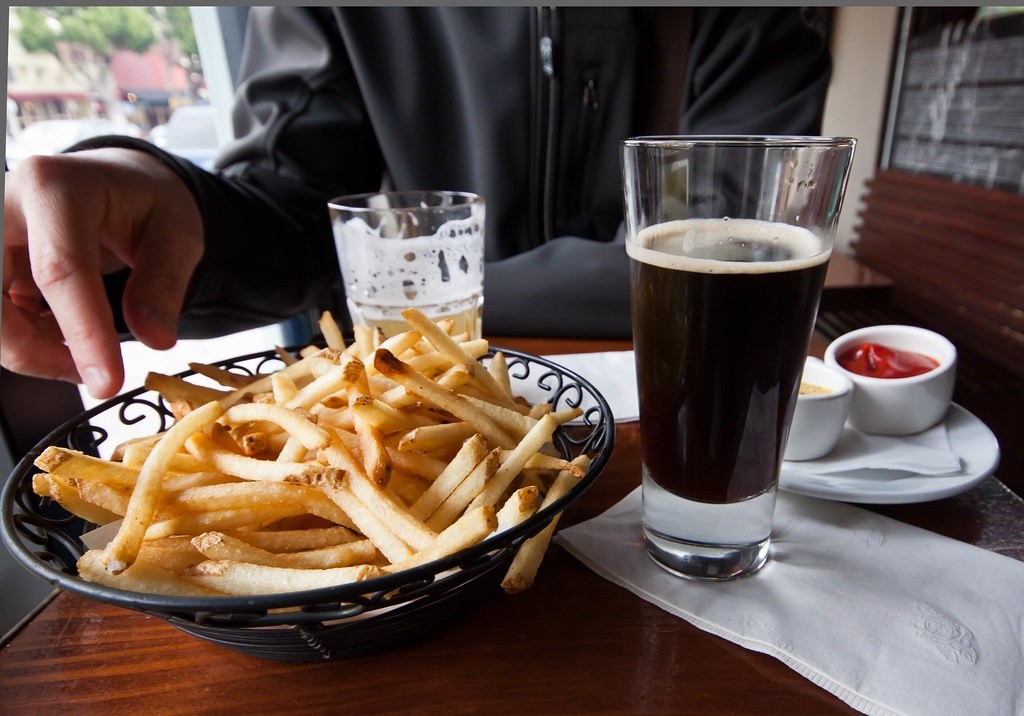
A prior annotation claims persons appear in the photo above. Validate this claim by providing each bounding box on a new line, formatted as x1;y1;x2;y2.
1;5;840;402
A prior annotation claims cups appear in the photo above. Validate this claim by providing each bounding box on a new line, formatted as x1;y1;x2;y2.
621;135;858;583
328;191;486;339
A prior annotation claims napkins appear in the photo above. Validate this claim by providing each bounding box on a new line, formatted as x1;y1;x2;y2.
484;350;641;425
783;418;963;478
553;481;1024;716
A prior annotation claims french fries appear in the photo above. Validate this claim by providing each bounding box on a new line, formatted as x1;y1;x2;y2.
31;310;592;613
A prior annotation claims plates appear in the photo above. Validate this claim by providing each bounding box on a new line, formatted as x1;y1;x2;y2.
778;401;1000;504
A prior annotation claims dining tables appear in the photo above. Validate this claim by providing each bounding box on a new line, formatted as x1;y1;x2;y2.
0;326;1024;716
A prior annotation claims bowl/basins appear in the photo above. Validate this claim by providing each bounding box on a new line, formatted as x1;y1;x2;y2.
824;325;957;436
783;365;855;461
